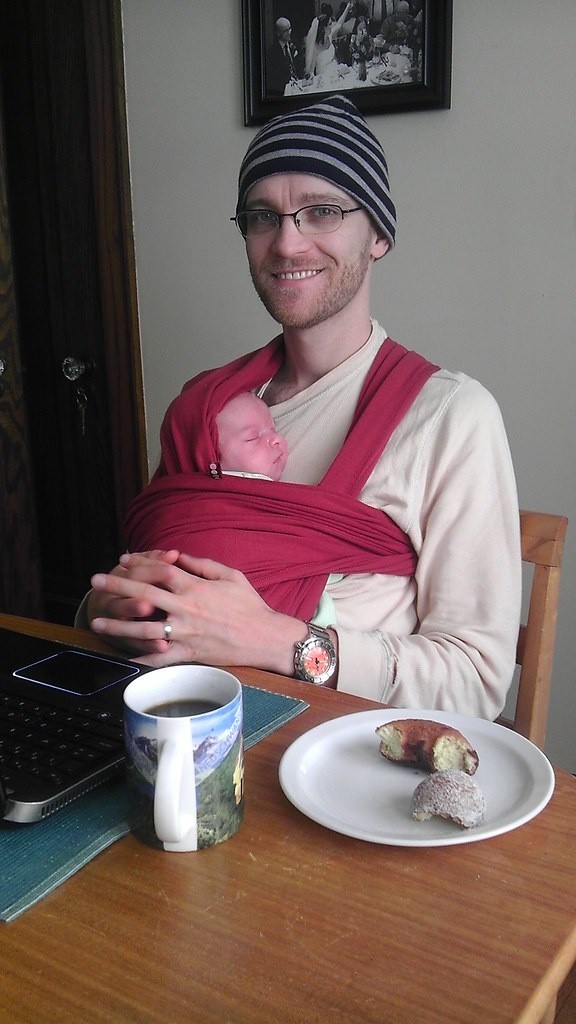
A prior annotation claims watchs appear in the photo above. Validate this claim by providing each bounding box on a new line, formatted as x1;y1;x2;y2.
291;619;337;687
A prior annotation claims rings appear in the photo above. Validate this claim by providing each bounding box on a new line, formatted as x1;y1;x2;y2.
161;619;172;643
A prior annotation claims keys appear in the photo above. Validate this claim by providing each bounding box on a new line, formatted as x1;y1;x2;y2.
71;377;89;436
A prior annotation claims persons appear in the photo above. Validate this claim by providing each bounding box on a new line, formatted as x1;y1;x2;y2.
262;0;431;93
125;381;307;669
65;91;527;725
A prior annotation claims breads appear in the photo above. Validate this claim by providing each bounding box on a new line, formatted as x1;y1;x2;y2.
372;719;484;826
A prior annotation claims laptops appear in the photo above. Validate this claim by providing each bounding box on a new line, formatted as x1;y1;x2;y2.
1;626;158;826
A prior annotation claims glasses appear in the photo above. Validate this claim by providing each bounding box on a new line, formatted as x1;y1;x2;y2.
230;204;366;239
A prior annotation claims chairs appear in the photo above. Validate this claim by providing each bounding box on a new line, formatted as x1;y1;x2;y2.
491;506;569;755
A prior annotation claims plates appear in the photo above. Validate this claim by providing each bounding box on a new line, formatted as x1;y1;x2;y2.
279;705;556;846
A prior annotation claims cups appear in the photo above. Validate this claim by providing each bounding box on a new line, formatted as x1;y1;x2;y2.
124;665;247;857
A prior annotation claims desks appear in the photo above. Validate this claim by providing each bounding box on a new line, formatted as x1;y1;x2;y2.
2;612;576;1023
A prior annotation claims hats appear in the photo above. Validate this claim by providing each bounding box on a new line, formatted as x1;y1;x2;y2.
238;95;395;263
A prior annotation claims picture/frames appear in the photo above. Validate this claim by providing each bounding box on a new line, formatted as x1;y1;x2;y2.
239;0;453;130
